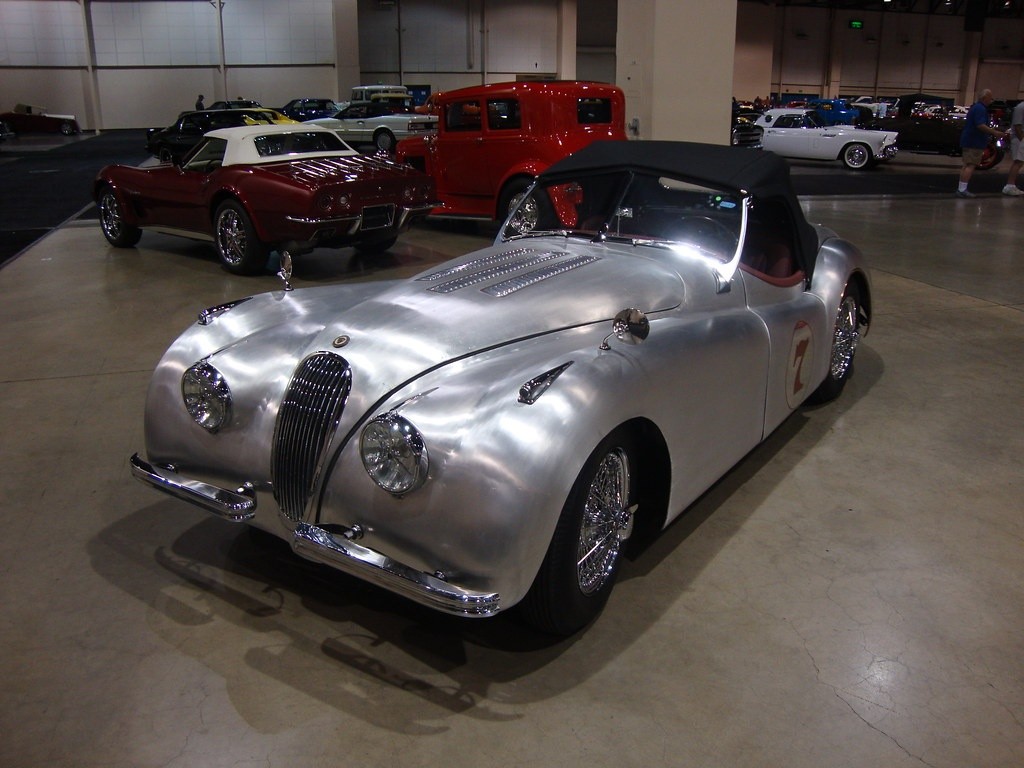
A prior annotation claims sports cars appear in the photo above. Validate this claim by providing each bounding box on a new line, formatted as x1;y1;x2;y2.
298;102;439;153
129;137;874;640
90;123;447;276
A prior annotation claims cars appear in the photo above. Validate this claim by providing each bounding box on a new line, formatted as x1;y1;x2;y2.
730;90;1011;172
143;84;409;165
395;80;630;235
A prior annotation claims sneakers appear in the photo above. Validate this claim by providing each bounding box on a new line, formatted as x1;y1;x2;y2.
955;189;976;199
1002;186;1024;196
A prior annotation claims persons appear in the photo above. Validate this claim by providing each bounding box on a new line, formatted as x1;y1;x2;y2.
1002;101;1024;195
195;95;205;111
956;89;1003;199
877;97;887;119
754;95;777;107
